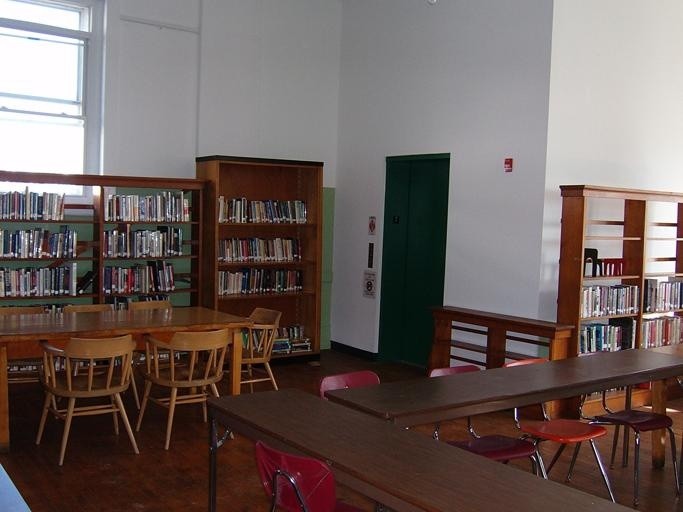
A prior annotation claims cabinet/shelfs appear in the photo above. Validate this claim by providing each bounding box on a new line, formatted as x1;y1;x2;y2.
557;184;683;420
0;170;213;395
195;155;325;362
428;306;576;420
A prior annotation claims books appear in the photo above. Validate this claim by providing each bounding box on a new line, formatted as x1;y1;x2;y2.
130;350;180;365
77;271;99;294
242;326;312;354
218;238;303;295
0;224;77;297
218;196;307;224
581;246;682;400
6;356;122;373
104;191;189;311
1;192;66;220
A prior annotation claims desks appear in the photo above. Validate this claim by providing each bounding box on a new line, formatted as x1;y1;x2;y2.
644;343;683;469
205;386;643;512
